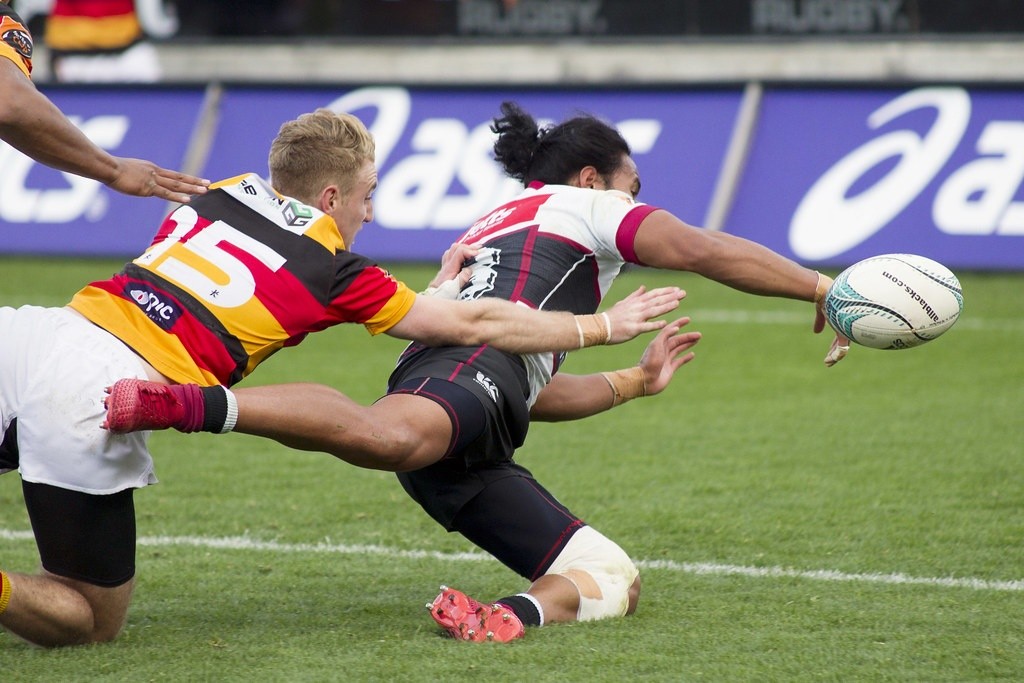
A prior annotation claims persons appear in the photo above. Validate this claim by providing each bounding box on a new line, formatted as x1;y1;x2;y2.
1;0;211;205
1;106;687;648
100;102;849;647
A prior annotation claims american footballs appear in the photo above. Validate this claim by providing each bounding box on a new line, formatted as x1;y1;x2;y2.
824;253;965;350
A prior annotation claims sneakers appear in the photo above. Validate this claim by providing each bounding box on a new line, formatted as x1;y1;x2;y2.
426;585;525;645
97;378;186;434
